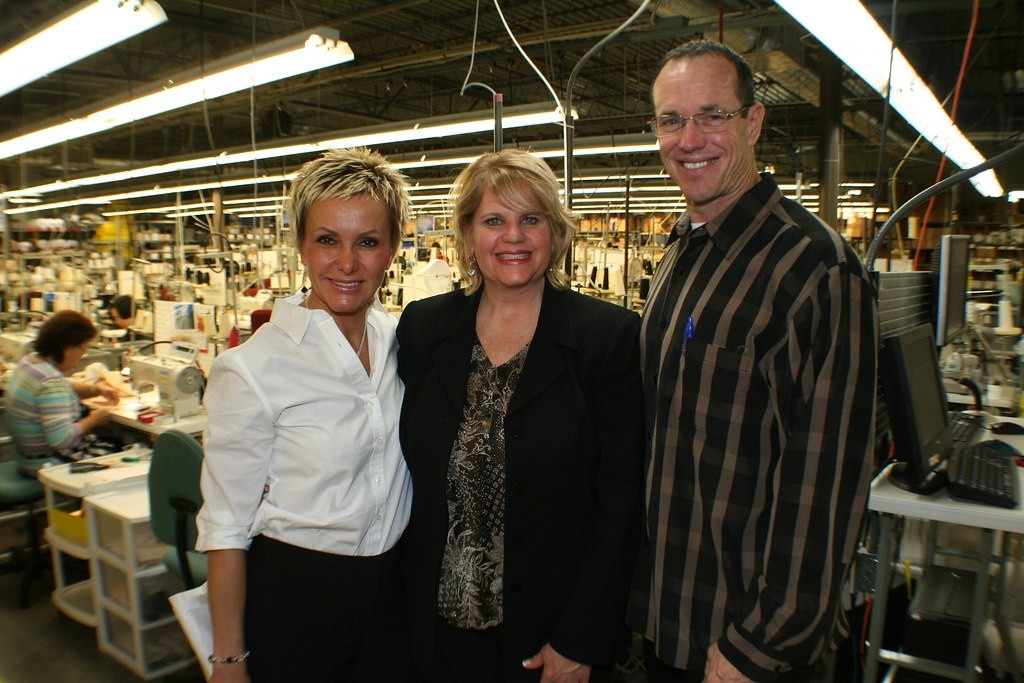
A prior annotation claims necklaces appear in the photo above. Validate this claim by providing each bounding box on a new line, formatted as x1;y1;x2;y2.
305;297;366;357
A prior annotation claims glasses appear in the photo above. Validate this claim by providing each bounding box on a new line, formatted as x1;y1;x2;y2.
647;106;748;137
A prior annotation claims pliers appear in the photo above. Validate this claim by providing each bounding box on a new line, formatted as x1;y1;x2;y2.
69;462;131;474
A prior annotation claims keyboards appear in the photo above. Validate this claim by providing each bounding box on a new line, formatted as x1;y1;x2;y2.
948;444;1019;510
947;411;984;450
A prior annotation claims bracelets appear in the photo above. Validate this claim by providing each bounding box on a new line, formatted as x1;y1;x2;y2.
91;385;96;396
209;651;249;664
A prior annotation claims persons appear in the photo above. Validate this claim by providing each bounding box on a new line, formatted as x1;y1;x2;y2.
260;148;646;683
194;149;413;683
109;295;139;338
626;38;879;683
7;311;119;512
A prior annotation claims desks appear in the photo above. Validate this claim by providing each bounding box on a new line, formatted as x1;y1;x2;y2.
857;378;1024;683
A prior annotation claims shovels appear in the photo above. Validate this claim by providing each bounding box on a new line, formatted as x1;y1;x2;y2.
121;452;153;462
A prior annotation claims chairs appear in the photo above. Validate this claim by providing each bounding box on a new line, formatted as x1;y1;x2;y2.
39;445;200;681
1;456;58;608
148;430;208;590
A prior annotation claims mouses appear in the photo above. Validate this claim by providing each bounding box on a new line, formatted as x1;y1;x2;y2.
991;422;1024;435
980;439;1019;455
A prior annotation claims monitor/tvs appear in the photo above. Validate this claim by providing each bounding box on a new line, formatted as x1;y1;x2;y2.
932;234;971;347
886;322;954;488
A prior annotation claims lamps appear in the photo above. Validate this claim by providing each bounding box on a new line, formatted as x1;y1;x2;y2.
0;24;354;161
3;100;579;199
0;0;169;100
773;0;1006;199
4;131;887;222
